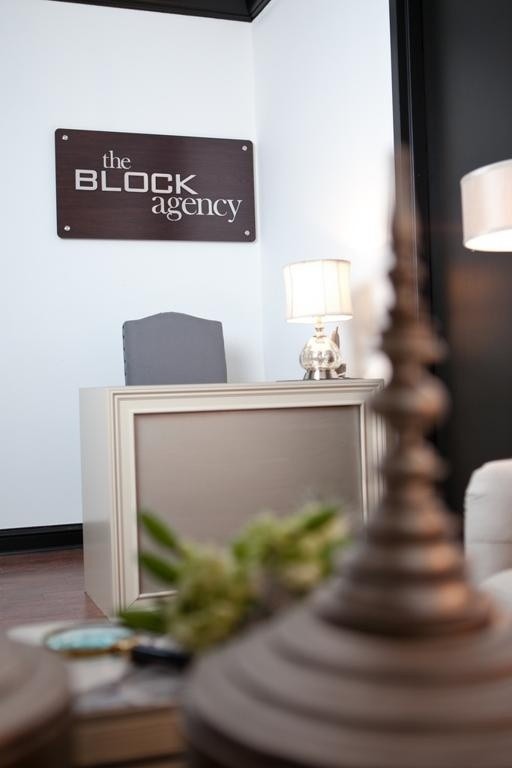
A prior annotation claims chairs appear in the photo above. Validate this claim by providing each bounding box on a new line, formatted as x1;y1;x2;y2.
117;309;228;390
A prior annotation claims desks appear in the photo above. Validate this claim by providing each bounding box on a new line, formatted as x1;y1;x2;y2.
76;379;385;629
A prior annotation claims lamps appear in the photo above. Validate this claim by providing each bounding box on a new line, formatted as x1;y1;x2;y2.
461;155;512;257
278;253;364;381
